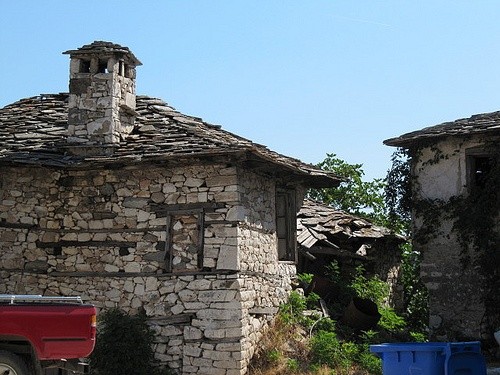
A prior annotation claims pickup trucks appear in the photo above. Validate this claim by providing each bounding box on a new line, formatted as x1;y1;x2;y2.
0;289;99;374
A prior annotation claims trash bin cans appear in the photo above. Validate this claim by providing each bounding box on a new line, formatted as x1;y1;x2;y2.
368;339;487;375
